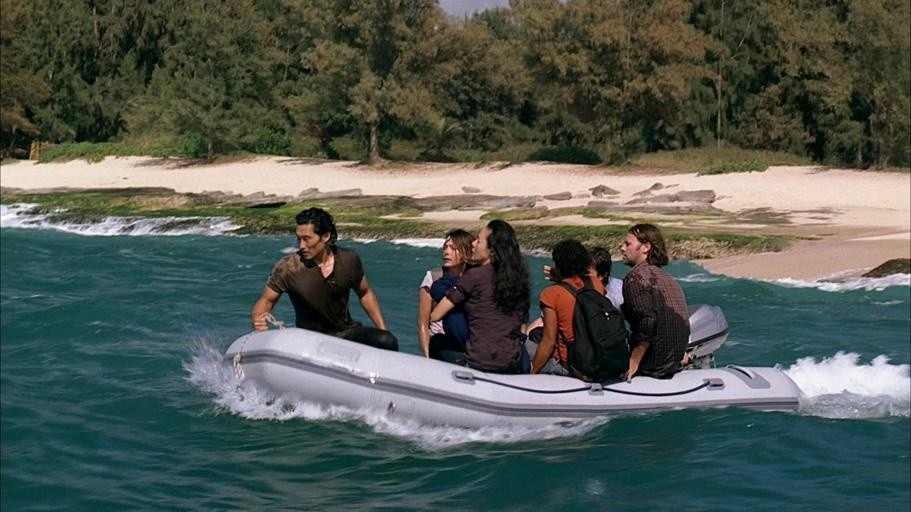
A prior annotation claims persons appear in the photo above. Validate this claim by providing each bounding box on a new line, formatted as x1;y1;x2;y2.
619;223;691;383
527;246;624;344
417;229;476;360
524;237;608;377
251;207;399;353
428;220;531;375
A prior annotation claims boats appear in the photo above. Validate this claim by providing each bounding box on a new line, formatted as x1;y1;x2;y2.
211;296;804;450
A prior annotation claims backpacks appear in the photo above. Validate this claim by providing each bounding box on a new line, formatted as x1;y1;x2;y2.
552;273;632;386
